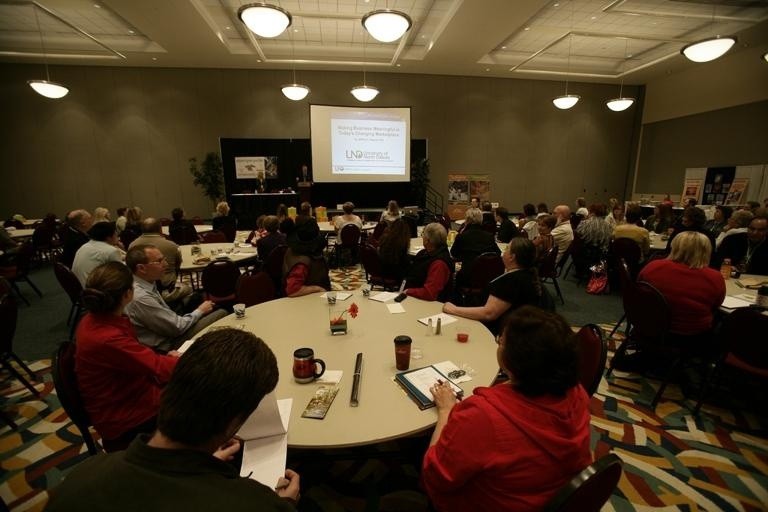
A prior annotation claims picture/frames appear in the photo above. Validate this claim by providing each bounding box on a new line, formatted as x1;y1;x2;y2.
448;174;492;206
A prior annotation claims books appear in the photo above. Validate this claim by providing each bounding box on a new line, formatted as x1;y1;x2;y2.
395;365;463;410
235;385;294;497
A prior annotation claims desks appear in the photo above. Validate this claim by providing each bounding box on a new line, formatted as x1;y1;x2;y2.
408;236;461;256
167;241;259;280
4;228;36;238
21;218;44;227
649;233;671;250
176;290;502;448
160;225;213;235
456;215;519;229
719;271;768;316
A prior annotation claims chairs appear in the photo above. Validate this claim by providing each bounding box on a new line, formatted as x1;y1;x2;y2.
234;269;277;309
51;339;96;456
554;244;575;286
32;220;69;262
204;231;228;243
359;241;407;291
0;292;41;397
200;256;241;309
333;223;360;265
190;216;204;225
53;262;88;328
537;245;565;304
0;243;44;307
20;213;56;266
607;281;713;416
317;221;384;237
539;453;625;512
571;322;607;397
458;253;506;306
609;266;636;341
158;217;174;226
390;218;410;237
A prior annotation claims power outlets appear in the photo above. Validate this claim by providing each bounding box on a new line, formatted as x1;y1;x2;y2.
493;203;500;209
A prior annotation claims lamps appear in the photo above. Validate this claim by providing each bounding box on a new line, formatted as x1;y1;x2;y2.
25;3;70;99
281;27;312;100
361;0;413;43
236;0;292;38
350;30;381;102
605;39;635;112
552;1;581;110
680;0;738;63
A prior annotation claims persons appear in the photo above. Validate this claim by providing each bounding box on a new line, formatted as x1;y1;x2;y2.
276;205;293;229
576;202;611;263
442;237;542;334
88;207;121;245
493;206;517;242
575;198;588;216
126;218;191;316
380;201;401;223
672;198;697;222
707;205;730;235
645;206;667;233
59;210;90;263
124;244;228;354
296;203;320;234
71;260;192;452
605;201;624;230
467;197;480;209
715;216;768;277
363;218;408;266
762;200;768;212
535;203;549;218
43;331;300;511
256;215;286;260
551;204;573;267
334;203;362;243
529;216;554;265
72;221;126;293
636;231;725;369
116;206;128;237
479;201;493;225
738;201;762;214
713;210;755;243
609;203;648;262
419;305;591;511
0;225;20;264
519;204;536;231
245;215;280;243
213;200;236;243
450;209;501;270
400;223;453;301
255;172;266;195
665;209;715;259
119;206;145;251
169;208;201;244
280;218;326;259
32;213;55;254
295;166;312;185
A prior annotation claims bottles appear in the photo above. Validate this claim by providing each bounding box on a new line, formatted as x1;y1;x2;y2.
721;258;731;280
435;318;441;336
425;319;433;336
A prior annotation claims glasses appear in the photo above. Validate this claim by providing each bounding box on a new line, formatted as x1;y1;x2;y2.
495;333;505;346
147;256;168;266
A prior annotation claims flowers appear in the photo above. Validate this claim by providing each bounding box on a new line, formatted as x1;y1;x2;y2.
328;303;358;325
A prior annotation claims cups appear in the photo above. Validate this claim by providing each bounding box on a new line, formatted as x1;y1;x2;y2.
326;292;337;305
292;346;326;386
394;336;412;370
457;327;470;342
233;304;246;318
190;241;241;256
362;284;371;298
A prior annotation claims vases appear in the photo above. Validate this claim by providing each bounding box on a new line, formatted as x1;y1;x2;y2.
328;307;349;335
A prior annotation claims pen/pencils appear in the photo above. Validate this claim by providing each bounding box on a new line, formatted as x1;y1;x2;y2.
436;377;463;402
275;483;288;488
